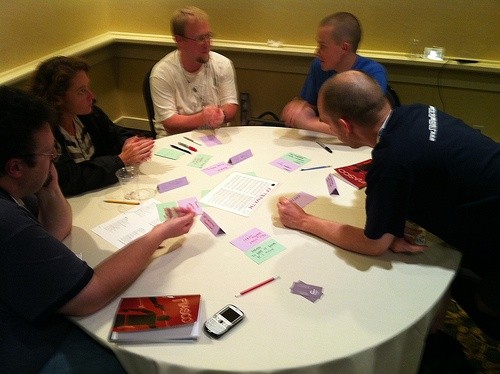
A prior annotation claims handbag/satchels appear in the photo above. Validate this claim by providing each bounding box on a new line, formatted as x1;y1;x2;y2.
246;112;286;127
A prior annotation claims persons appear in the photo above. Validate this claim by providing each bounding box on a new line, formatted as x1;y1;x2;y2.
149;5;239;139
277;69;500;364
30;55;155;198
279;11;387;137
0;83;197;374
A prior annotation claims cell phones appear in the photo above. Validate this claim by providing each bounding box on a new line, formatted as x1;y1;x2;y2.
204;304;244;339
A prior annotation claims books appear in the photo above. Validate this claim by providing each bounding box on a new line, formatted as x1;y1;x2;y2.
108;294;201;344
332;159;372;190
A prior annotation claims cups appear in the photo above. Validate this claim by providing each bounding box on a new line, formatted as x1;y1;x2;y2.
115;166;142;200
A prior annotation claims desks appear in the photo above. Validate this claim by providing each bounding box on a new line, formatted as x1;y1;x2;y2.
59;126;462;374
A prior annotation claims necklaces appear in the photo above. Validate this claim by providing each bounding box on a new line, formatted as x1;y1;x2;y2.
180;61;208;110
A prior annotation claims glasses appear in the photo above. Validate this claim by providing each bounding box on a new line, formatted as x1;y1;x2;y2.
32;146;62;163
182;31;215;44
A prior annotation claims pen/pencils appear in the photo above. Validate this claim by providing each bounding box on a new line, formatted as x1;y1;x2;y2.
104;200;140;205
301;166;331;171
235;275;279;297
170;145;190;154
184;137;200;146
178;142;197;152
316;140;332;153
